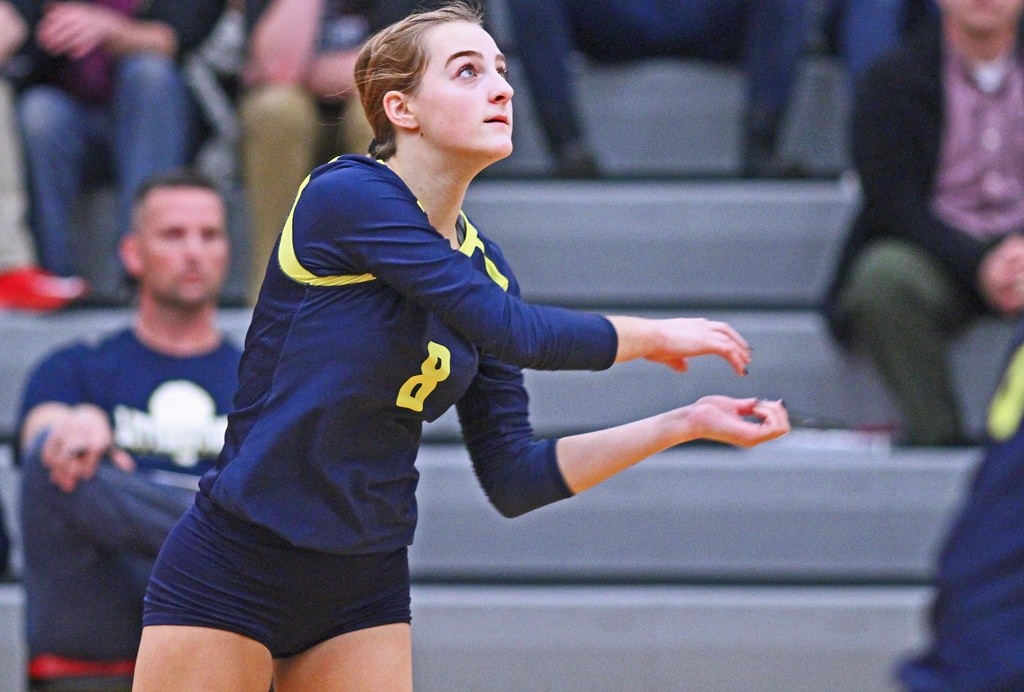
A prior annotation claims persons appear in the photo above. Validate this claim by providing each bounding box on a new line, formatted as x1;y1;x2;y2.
11;169;242;690
0;0;910;309
821;1;1024;691
132;0;791;692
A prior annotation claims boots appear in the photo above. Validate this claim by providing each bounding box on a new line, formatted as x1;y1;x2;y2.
739;105;809;181
540;111;603;178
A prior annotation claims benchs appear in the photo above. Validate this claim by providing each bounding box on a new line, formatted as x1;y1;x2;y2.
1;55;1024;692
